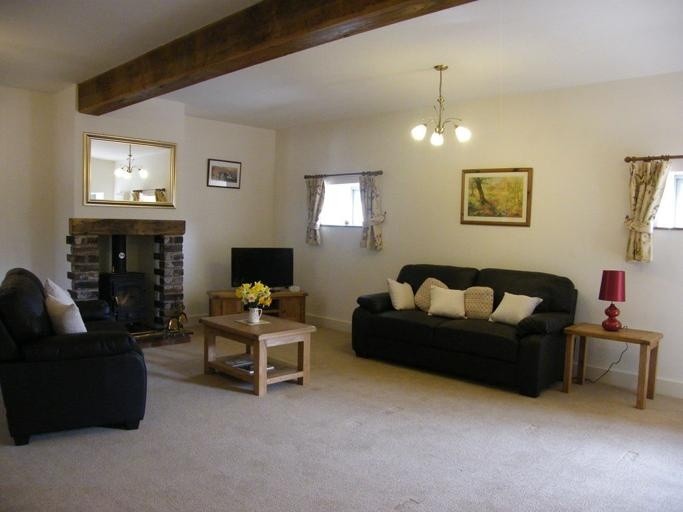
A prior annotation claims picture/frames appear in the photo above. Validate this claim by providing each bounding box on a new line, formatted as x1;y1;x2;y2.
207;159;242;189
460;167;533;227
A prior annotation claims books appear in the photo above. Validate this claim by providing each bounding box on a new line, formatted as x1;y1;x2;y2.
228;355;274;374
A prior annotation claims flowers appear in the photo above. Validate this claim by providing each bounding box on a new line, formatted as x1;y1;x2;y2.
235;281;273;308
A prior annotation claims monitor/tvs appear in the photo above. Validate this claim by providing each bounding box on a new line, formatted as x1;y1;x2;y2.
231;247;294;288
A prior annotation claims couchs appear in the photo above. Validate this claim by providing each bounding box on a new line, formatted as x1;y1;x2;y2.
0;267;147;446
351;263;578;398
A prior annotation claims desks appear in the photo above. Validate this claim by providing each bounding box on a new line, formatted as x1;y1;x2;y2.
562;322;663;410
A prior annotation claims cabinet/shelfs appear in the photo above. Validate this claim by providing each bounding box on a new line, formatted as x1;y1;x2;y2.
206;289;310;324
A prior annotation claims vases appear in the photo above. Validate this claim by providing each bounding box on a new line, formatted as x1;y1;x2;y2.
248;307;263;323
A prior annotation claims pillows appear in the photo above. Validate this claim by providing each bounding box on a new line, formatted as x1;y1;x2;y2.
45;277;74;304
45;293;87;334
386;277;417;311
488;291;543;327
428;284;467;320
414;278;449;312
464;286;494;320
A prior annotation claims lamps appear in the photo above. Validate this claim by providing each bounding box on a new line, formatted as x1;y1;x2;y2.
598;270;626;331
410;64;472;147
114;144;148;180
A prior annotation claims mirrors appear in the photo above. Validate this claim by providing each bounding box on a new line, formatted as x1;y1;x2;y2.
82;131;178;209
